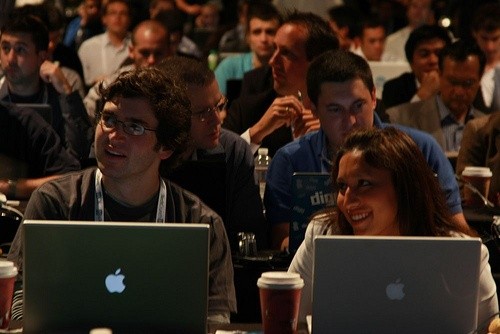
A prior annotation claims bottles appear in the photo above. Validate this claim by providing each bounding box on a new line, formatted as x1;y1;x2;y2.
254;147;273;214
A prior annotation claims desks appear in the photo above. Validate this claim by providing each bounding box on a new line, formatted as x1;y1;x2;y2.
0;175;500;334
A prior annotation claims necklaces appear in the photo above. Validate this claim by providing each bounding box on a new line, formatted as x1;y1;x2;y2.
93;168;168;224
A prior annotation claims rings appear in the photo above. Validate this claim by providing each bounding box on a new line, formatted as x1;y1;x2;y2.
284;106;289;114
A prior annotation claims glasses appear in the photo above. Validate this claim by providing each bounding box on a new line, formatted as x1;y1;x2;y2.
96;112;161;136
191;96;228;122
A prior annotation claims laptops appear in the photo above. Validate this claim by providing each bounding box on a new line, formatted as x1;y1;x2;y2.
306;235;482;334
289;172;340;263
22;218;210;334
365;61;414;101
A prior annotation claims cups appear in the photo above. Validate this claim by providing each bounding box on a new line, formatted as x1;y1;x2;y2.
0;261;18;333
462;167;493;209
256;271;305;334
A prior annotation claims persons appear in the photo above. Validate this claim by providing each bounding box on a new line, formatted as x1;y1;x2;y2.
79;2;140;91
29;1;499;60
1;59;97;187
0;12;87;137
259;49;479;261
377;22;487;118
152;55;264;273
214;1;284;98
285;125;500;334
81;19;172;118
385;35;486;159
5;65;239;326
455;111;500;214
216;10;393;163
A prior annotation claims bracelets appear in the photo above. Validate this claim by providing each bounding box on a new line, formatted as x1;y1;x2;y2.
5;179;17;198
